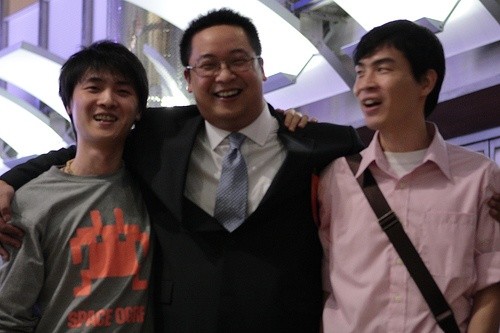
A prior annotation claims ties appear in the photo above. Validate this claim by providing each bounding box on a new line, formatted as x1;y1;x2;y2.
213;131;248;233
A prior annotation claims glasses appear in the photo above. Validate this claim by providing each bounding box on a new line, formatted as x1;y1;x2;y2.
186;54;259;78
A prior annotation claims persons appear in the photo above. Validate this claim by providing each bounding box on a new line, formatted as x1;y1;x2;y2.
0;7;500;333
0;38;318;333
313;20;499;332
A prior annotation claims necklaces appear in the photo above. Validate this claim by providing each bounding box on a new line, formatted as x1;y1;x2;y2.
64;158;76;176
